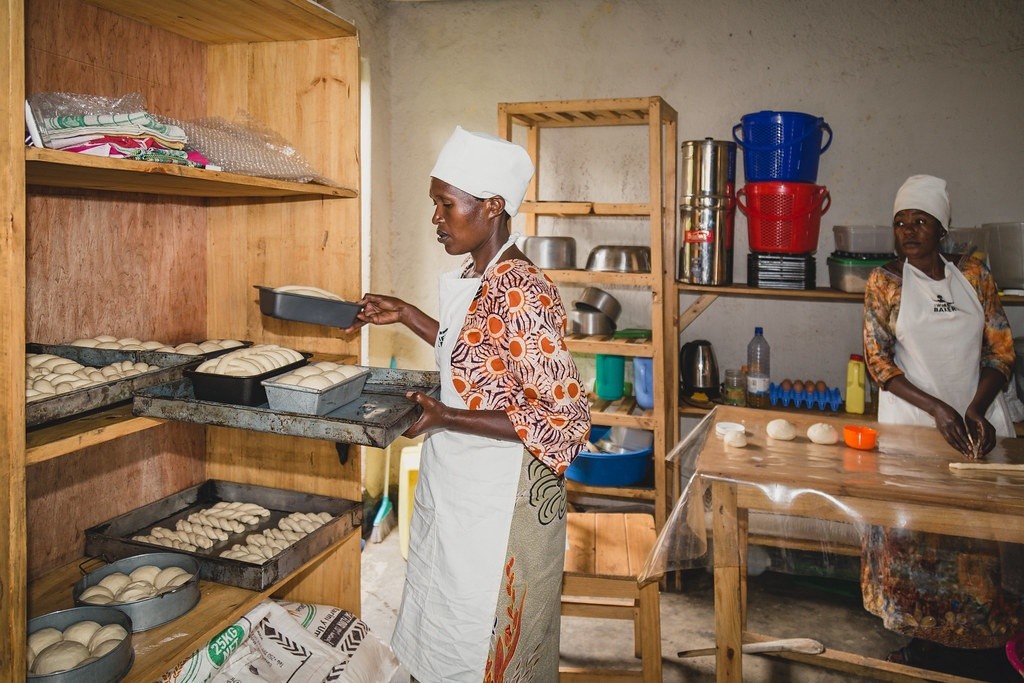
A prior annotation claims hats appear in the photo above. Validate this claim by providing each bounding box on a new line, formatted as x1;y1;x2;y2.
430;125;536;217
892;174;951;232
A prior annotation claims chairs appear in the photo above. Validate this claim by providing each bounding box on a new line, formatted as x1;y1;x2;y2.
559;513;667;683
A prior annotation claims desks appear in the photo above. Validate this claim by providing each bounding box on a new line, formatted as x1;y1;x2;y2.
636;404;1024;683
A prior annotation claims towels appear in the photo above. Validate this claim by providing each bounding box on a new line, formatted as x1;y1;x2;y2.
37;110;225;172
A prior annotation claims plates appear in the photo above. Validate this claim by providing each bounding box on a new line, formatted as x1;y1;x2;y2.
715;422;746;436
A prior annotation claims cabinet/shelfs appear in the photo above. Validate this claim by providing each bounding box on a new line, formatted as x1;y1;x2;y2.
0;0;362;683
496;95;678;683
675;280;1024;569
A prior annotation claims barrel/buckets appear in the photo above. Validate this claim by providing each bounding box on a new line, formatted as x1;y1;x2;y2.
678;209;736;286
681;136;737;208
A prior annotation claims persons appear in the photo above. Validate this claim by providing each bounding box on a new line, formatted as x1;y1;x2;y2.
862;173;1024;683
344;124;591;683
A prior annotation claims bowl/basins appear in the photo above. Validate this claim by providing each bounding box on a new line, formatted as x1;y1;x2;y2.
523;237;576;270
564;426;654;487
585;245;651;273
572;311;612;335
843;425;878;449
575;287;622;331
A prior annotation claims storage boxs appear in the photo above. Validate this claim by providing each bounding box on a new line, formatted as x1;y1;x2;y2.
827;257;892;293
832;224;894;253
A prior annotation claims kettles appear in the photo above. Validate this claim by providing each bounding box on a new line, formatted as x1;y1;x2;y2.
680;340;721;397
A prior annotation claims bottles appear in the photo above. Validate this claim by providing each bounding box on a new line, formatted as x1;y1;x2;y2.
846;354;866;414
746;327;771;408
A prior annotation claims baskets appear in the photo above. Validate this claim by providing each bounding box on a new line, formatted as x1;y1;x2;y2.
732;110;833;183
564;426;653;487
736;181;831;255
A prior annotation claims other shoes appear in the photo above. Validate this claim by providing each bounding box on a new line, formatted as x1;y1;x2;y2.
886;646;943;666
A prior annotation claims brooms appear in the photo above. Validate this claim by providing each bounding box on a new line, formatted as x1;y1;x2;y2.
370;353;397;544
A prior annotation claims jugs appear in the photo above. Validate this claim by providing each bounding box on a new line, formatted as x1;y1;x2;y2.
633;358;653;409
594;354;625;400
